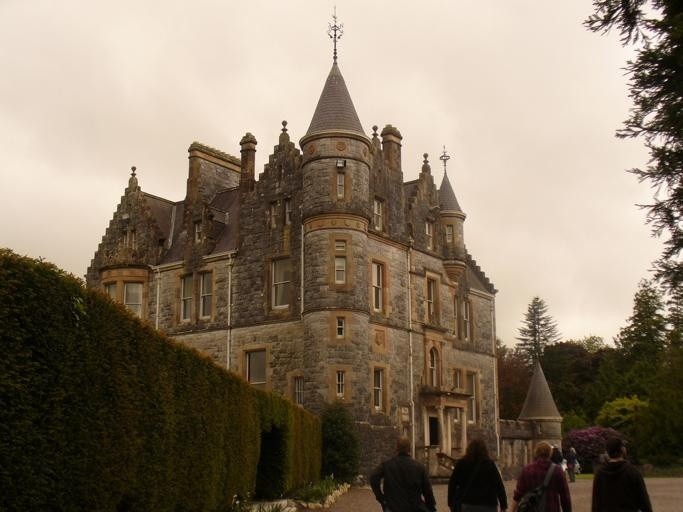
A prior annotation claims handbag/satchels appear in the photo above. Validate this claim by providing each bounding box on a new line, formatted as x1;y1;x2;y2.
517;486;546;511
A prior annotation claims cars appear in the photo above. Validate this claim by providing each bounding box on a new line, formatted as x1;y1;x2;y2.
561;458;581;476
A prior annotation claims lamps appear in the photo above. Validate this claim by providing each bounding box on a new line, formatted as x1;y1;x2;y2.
336;160;346;167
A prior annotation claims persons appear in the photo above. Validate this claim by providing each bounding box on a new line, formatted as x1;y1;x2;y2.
550;443;562;466
508;441;572;512
589;436;653;512
368;437;437;512
565;447;577;483
445;437;508;512
